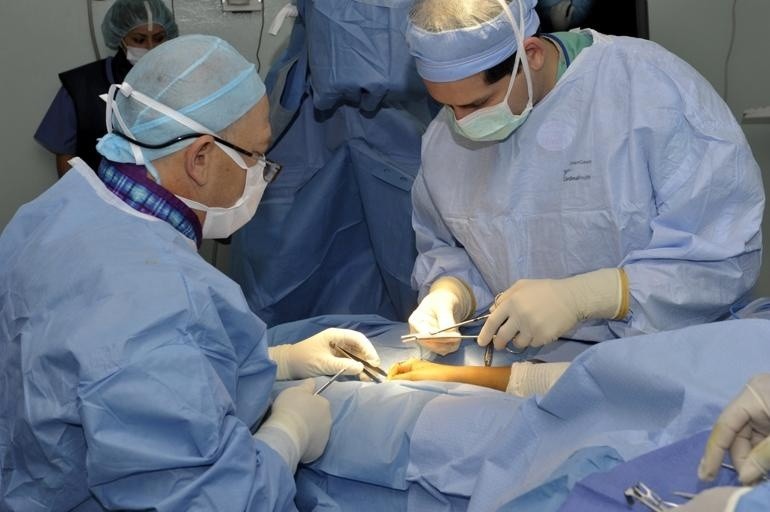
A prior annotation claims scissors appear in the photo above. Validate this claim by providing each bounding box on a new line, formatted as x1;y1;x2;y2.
400;291;528;355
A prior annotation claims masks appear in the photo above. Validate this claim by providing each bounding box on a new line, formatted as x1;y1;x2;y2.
189;142;268;239
120;39;148;66
444;44;533;142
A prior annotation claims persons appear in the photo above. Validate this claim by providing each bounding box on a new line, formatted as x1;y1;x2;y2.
405;0;766;350
0;33;380;512
386;357;571;401
672;374;770;512
33;0;179;179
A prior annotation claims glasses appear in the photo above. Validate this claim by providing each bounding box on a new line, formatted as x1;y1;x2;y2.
176;134;281;183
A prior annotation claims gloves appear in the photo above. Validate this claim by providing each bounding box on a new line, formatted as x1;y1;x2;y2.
697;373;770;485
252;379;331;476
270;326;380;381
478;267;621;346
665;486;752;512
407;277;472;356
